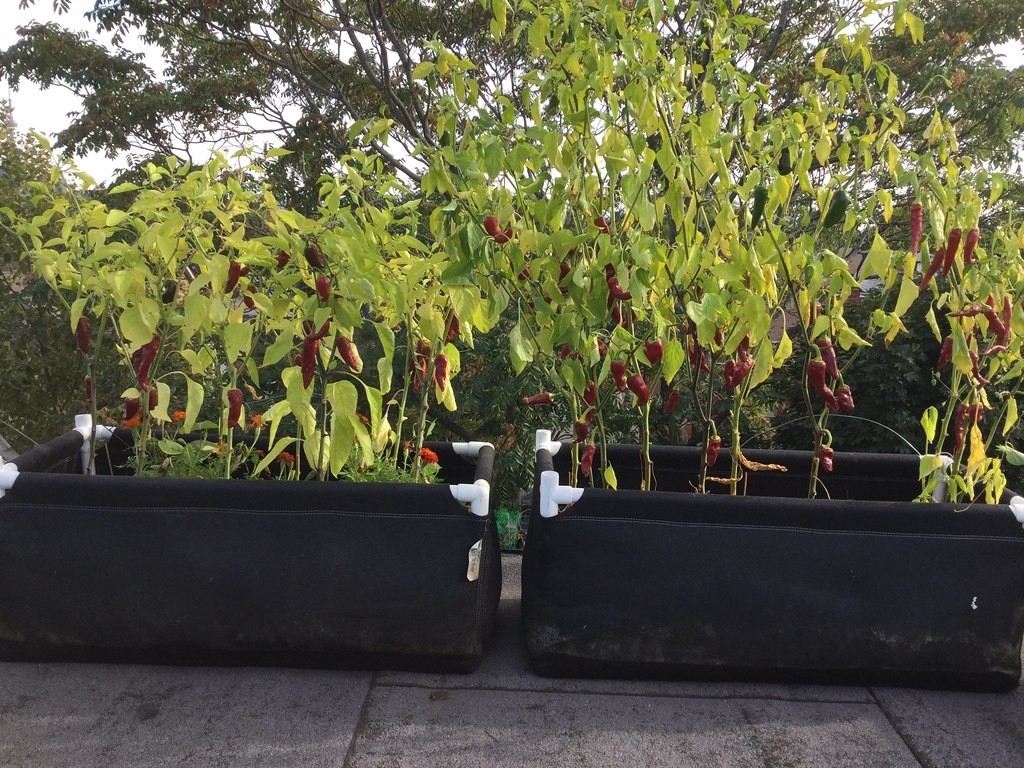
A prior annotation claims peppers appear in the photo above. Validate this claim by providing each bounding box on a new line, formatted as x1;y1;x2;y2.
74;200;1012;477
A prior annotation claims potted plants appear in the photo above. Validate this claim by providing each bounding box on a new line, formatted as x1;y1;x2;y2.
0;0;1024;692
